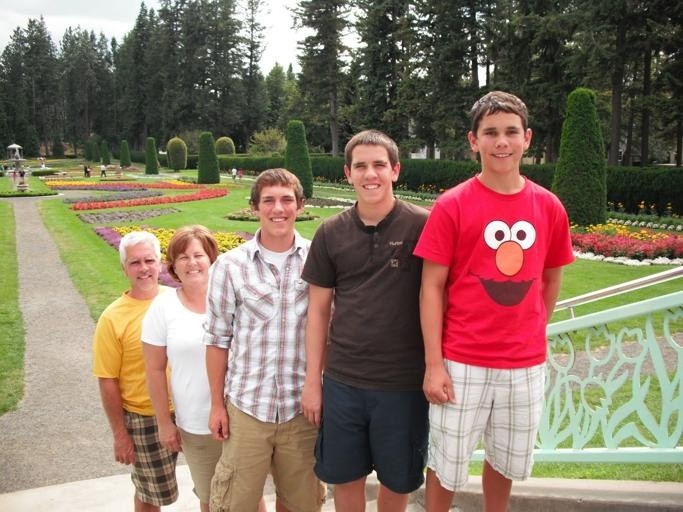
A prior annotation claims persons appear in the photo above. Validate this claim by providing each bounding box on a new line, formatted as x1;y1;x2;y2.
99;163;106;176
230;165;237;182
83;164;88;178
18;168;25;180
12;169;17;183
87;165;92;178
198;167;334;511
92;230;179;511
410;89;575;512
237;168;242;177
139;225;263;510
298;130;433;511
